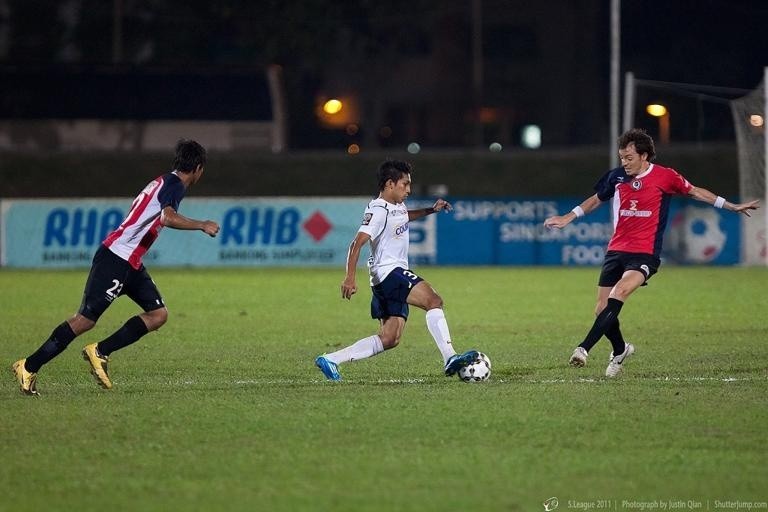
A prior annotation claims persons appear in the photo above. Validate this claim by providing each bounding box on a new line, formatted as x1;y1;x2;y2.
313;157;479;383
9;137;220;395
541;124;761;380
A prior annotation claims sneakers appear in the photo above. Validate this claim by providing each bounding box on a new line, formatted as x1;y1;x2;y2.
11;359;41;398
443;350;478;376
82;343;111;389
606;342;634;377
314;356;340;380
568;347;588;367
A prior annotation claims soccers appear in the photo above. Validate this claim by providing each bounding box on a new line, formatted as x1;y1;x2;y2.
458;351;491;383
667;204;726;264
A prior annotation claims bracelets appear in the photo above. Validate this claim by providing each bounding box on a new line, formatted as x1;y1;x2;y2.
570;202;585;220
711;193;727;213
